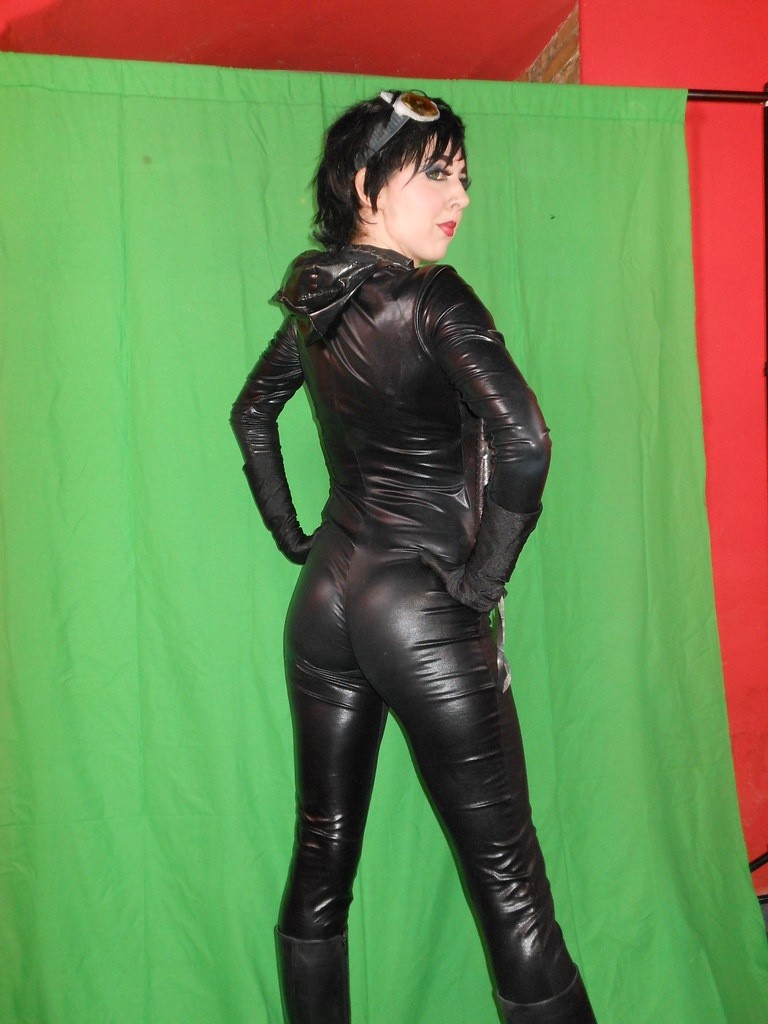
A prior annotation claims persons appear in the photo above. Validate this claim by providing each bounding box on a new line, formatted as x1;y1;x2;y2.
227;88;598;1024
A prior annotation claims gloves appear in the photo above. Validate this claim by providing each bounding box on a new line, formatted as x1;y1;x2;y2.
242;448;317;564
419;483;543;611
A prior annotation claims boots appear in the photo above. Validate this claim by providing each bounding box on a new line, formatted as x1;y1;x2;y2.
492;962;600;1024
273;924;352;1024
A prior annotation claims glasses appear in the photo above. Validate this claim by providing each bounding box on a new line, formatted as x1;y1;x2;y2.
380;86;452;123
